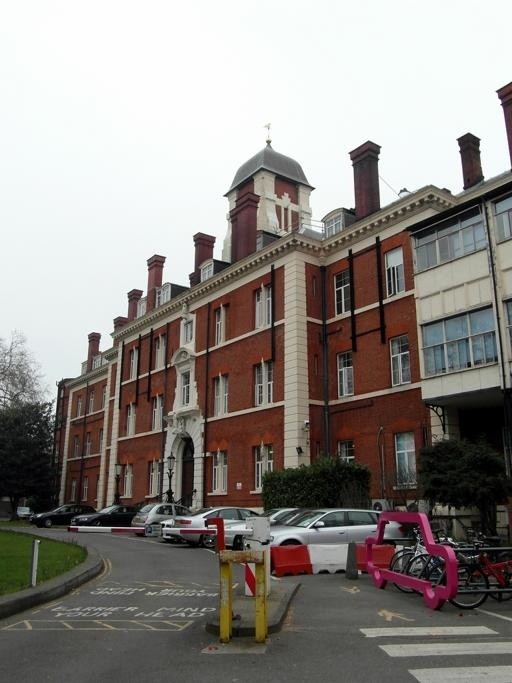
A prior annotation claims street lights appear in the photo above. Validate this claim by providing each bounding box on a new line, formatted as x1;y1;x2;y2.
114;460;123;504
166;452;175;503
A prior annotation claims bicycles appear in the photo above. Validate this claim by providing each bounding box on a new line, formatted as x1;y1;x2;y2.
389;527;512;610
